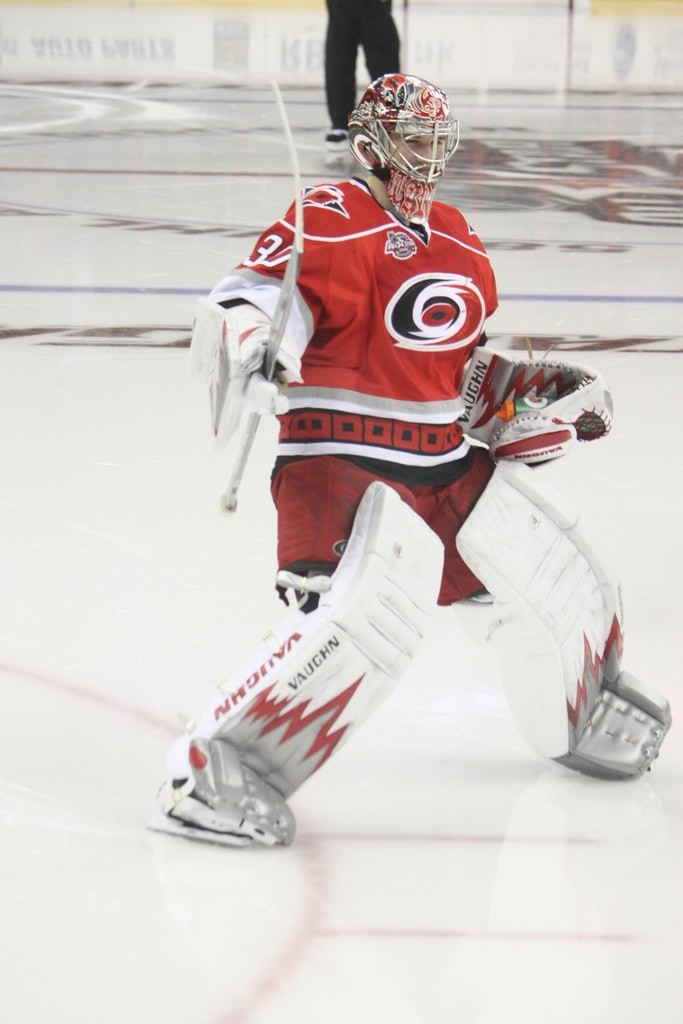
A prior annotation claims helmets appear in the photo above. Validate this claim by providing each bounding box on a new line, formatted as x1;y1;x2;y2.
348;73;461;182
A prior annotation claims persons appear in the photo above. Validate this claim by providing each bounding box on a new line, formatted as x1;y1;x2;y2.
324;0;405;150
147;73;672;848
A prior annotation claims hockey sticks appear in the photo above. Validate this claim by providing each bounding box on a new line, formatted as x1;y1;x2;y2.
222;76;308;515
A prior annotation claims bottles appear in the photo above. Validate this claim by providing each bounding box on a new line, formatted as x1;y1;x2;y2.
496;398;558;422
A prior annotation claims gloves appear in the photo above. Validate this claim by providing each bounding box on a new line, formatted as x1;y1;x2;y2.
190;297;303;442
457;347;613;448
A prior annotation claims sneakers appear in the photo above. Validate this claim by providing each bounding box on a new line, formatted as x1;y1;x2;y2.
324;129;352;172
158;734;295;848
